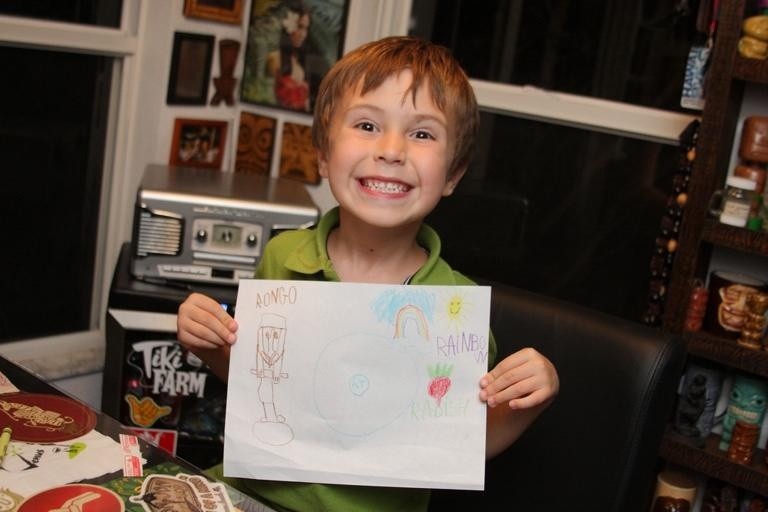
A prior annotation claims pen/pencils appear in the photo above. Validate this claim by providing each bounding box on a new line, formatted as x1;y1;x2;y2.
0;427;12;464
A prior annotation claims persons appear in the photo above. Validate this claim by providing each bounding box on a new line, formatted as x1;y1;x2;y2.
177;36;559;512
266;8;330;113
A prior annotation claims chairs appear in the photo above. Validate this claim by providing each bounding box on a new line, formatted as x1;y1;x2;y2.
428;278;686;510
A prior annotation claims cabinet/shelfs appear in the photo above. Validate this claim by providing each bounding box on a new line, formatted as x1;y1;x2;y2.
101;242;238;472
661;0;768;512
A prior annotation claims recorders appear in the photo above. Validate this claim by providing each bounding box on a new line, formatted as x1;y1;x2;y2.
130;162;322;288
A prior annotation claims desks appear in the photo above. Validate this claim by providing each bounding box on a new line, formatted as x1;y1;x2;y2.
0;356;292;511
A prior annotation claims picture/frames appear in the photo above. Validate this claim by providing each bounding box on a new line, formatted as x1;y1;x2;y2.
166;1;351;171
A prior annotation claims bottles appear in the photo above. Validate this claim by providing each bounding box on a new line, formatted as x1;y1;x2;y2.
708;176;756;229
